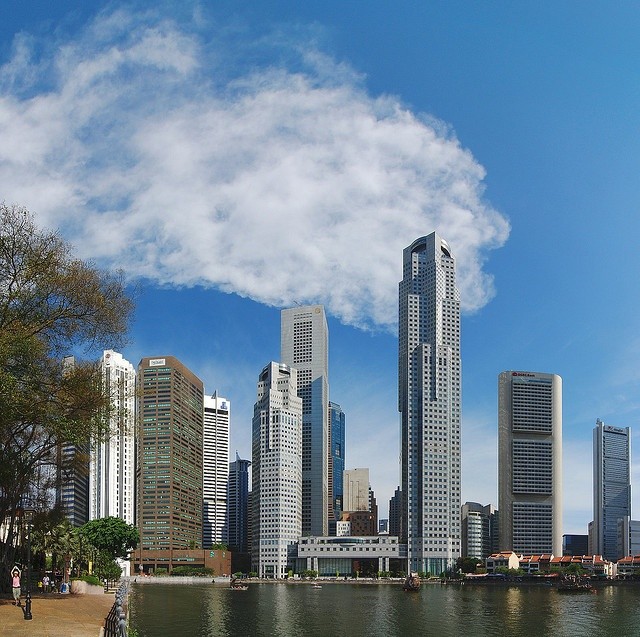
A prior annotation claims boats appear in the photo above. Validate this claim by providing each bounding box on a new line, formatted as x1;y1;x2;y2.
310;583;322;589
403;572;420;591
558;573;592;591
230;578;249;590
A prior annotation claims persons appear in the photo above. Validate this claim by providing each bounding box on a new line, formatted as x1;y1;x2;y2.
10;566;21;606
37;579;43;594
43;574;49;593
